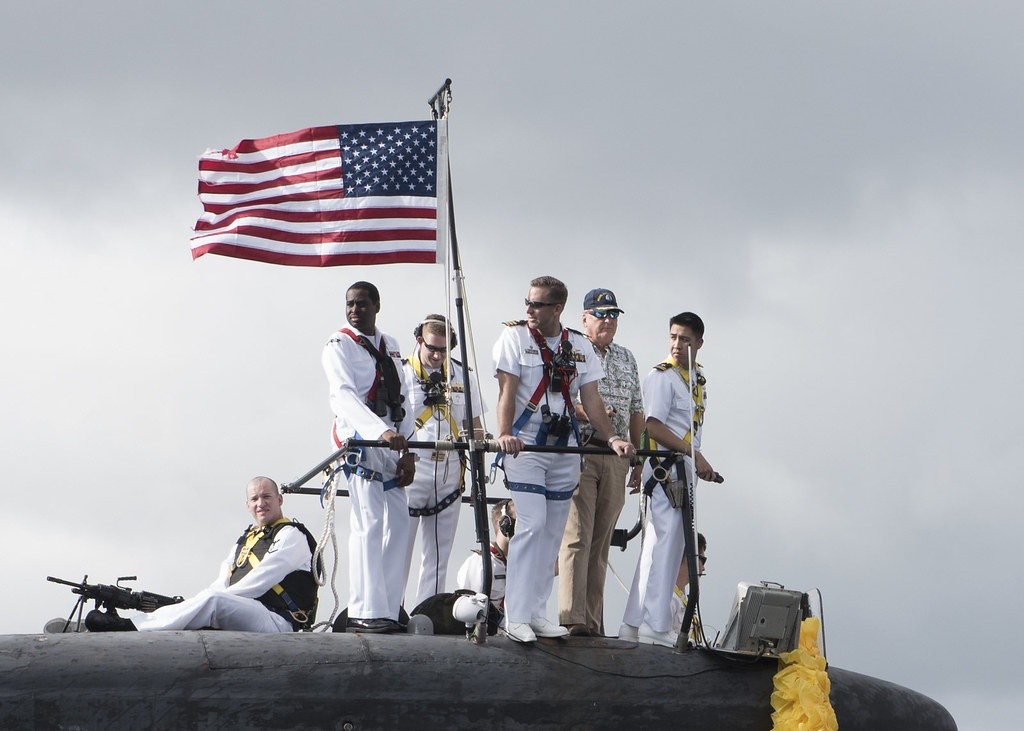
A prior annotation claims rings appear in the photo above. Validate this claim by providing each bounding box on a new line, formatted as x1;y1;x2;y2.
632;448;636;454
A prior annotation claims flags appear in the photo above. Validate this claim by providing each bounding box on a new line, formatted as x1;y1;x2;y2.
192;119;446;267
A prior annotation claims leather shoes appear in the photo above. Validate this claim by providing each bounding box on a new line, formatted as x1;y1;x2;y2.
345;618;393;633
385;620;401;632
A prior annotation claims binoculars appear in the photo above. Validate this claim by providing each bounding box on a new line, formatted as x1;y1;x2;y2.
535;413;572;453
375;380;404;423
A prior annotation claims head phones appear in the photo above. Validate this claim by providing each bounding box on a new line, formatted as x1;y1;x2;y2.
413;325;458;351
499;500;515;537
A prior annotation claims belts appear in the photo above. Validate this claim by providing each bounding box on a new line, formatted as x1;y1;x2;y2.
409;449;460;461
512;421;575;439
579;432;610;448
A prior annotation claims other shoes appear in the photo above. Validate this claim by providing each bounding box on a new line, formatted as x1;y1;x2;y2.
529;617;568;637
43;617;88;633
567;622;590;637
617;623;639;643
85;607;134;631
638;622;696;648
504;622;538;642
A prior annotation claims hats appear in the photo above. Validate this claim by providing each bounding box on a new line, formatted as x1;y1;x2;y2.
583;288;624;314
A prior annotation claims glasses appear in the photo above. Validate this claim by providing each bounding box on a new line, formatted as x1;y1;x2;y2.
590;311;620;319
524;298;563;310
423;338;446;354
698;554;707;566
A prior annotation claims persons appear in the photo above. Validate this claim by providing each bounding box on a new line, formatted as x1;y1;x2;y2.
457;499;517;637
324;281;413;632
399;315;485;607
670;532;707;647
45;477;322;632
559;288;642;636
492;276;637;643
618;312;716;648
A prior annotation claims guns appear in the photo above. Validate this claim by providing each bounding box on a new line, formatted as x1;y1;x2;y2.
46;574;187;633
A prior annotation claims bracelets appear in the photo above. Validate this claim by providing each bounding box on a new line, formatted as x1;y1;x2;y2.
636;461;642;467
607;436;620;448
